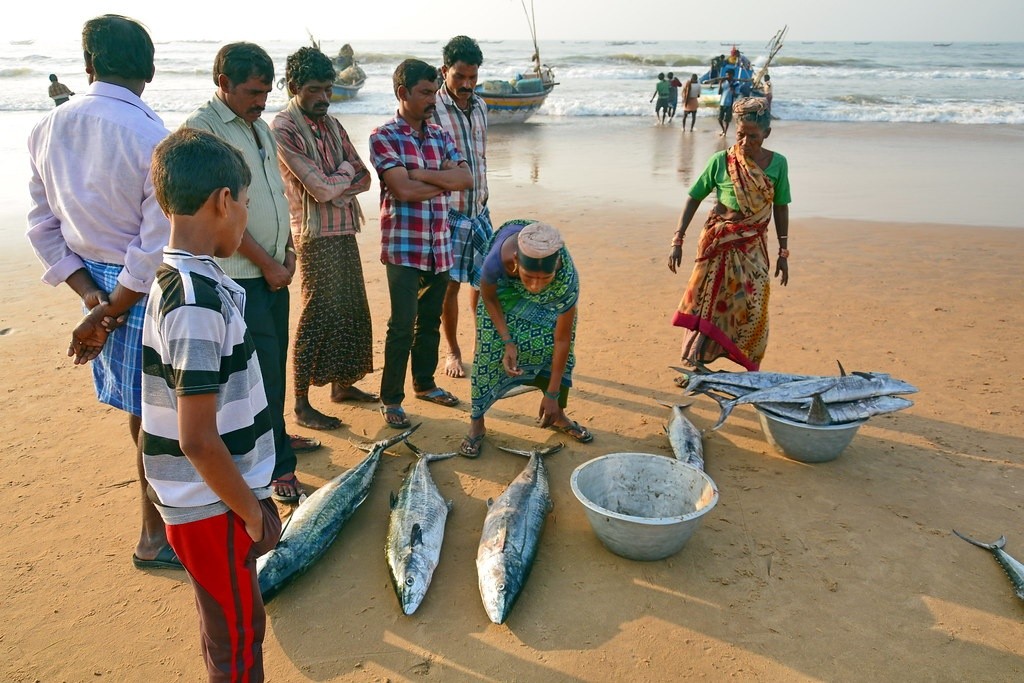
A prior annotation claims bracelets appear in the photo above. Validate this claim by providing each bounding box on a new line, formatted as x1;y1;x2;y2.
500;332;513;344
671;238;684;247
778;248;789;258
544;391;560;400
673;230;686;238
778;236;788;239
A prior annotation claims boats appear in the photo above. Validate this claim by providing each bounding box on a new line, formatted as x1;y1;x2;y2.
472;0;561;126
696;22;790;109
305;27;371;102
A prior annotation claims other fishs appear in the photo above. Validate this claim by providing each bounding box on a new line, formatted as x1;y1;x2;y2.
660;402;705;473
952;528;1024;601
383;439;460;615
667;356;920;431
475;440;564;623
256;422;424;606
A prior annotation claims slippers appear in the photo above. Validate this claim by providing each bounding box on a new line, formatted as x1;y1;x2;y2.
549;417;594;443
132;542;187;571
458;428;487;459
379;403;412;429
671;369;704;388
346;392;380;402
414;387;460;407
269;472;309;502
286;434;322;455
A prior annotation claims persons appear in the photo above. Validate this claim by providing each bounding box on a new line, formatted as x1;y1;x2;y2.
369;59;458;427
459;218;592;457
667;97;792;389
179;43;321;504
650;72;682;126
429;36;494;377
48;74;75;107
27;14;183;570
717;68;740;137
764;75;773;103
141;129;283;683
269;47;380;431
682;74;701;132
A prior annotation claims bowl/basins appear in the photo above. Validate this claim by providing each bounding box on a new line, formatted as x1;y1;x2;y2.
568;451;718;561
750;403;863;463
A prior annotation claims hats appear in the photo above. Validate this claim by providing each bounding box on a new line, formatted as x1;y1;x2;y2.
515;222;566;260
729;96;770;118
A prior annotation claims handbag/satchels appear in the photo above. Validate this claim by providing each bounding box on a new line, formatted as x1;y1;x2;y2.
671;78;682;87
